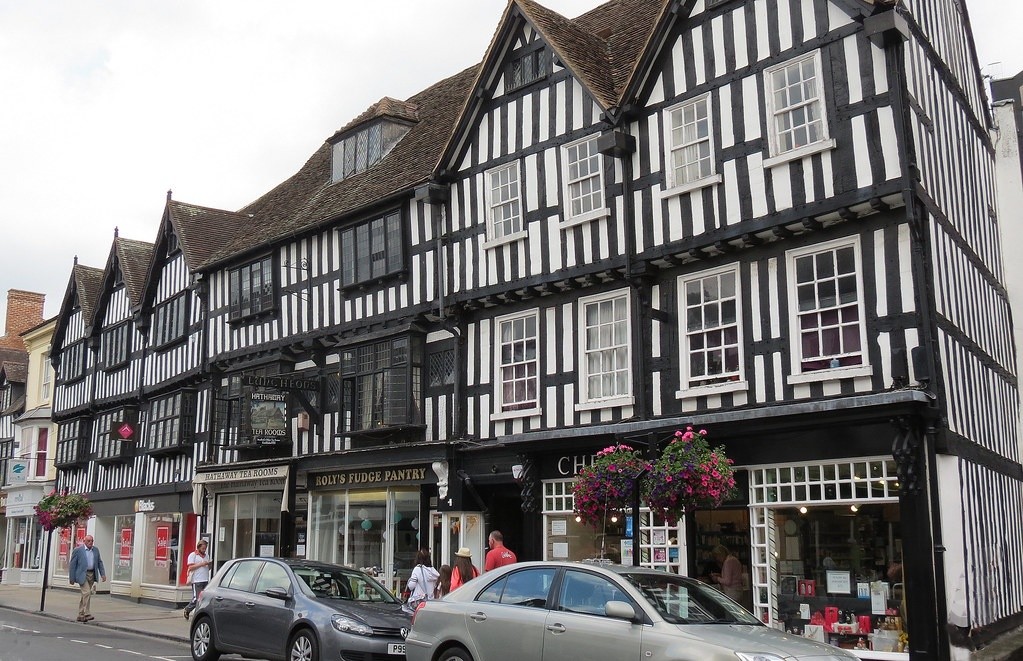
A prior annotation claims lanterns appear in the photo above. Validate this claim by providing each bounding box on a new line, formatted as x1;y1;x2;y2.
393;511;402;523
383;531;386;539
358;508;368;520
361;519;373;532
411;518;419;529
339;524;345;536
416;531;419;540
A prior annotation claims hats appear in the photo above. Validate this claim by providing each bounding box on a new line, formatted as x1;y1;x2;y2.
455;547;473;558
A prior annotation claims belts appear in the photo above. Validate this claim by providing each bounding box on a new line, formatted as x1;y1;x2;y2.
87;570;94;572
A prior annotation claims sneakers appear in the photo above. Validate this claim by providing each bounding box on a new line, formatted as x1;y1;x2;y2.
85;613;94;620
183;608;189;620
77;616;87;622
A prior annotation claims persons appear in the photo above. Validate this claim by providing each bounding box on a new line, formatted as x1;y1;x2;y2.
408;548;440;600
449;547;481;593
433;565;452;599
888;553;902;582
183;540;213;620
485;531;516;572
69;535;106;623
710;545;744;606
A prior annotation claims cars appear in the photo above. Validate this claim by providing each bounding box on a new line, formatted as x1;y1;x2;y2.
403;560;863;661
190;554;416;661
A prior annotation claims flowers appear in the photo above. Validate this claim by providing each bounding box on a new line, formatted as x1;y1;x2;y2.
33;489;94;532
571;428;733;528
